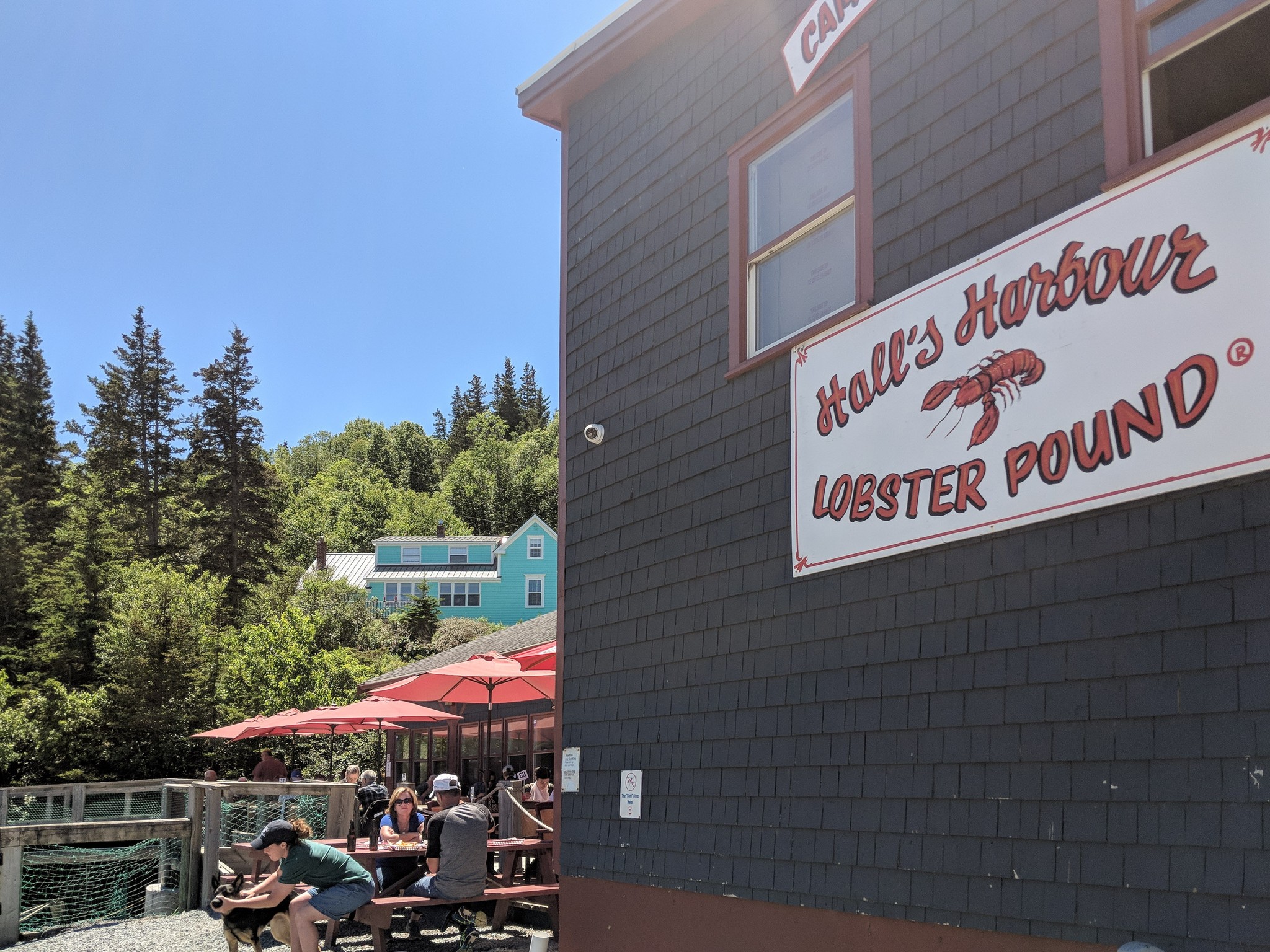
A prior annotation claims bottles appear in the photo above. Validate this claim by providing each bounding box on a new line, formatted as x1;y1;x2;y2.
369;819;379;851
347;820;357;852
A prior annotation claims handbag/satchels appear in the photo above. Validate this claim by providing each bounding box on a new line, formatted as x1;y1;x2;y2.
476;796;498;813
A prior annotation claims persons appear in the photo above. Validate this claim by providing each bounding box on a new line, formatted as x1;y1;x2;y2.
211;818;376;952
204;749;554;815
403;773;495;952
375;787;425;944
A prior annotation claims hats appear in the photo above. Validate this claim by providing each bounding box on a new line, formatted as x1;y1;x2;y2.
428;772;461;798
250;820;293;849
291;770;302;778
503;765;514;773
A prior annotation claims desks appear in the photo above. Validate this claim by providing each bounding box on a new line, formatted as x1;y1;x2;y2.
230;836;559;952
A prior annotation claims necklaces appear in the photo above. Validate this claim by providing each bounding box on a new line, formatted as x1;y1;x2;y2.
398;821;408;834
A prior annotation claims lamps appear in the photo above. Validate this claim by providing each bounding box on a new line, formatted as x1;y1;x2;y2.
365;586;372;590
497;576;502;578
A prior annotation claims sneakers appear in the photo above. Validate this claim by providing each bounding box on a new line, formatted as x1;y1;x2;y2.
456;927;479;952
453;908;487;928
406;920;422;940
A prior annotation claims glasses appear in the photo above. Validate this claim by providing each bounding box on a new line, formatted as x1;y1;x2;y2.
489;779;495;783
395;798;412;806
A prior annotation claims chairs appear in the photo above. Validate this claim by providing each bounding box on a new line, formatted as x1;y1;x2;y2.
520;800;554;886
360;798;392;837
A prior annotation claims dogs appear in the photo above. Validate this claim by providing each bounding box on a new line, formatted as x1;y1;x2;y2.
210;871;299;952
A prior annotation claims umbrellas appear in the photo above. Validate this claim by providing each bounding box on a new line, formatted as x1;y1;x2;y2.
366;640;556;810
189;696;464;785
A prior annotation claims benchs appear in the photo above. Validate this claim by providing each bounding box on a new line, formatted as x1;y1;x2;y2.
219;873;271;891
361;883;560;952
